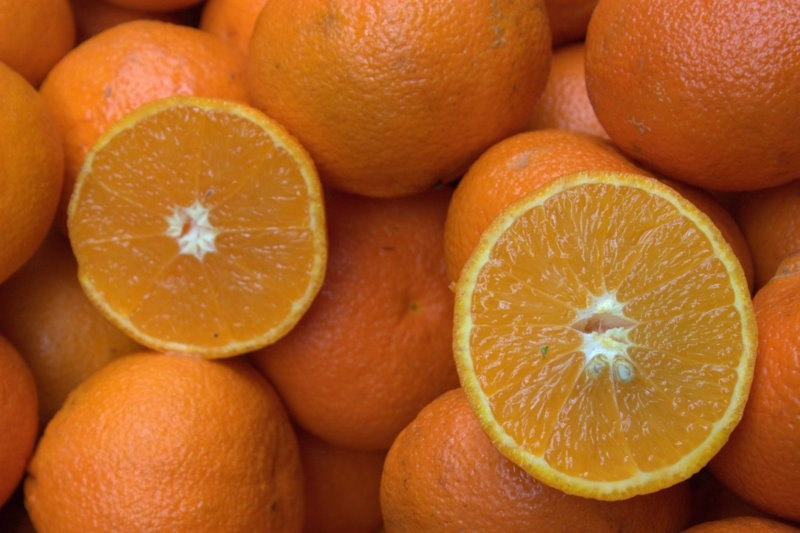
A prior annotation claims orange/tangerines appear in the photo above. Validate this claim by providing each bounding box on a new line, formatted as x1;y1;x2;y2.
0;0;800;533
